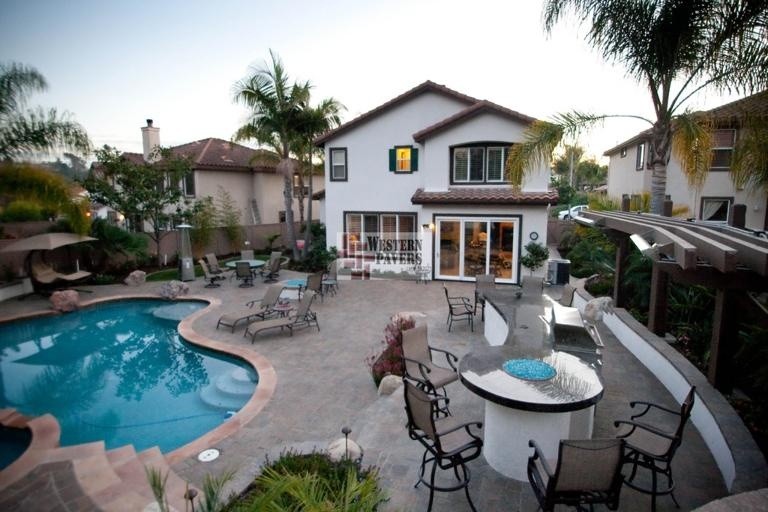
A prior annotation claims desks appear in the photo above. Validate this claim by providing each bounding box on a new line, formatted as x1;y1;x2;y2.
454;287;605;483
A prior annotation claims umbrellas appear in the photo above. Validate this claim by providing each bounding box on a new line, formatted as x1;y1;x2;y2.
0;230;99;252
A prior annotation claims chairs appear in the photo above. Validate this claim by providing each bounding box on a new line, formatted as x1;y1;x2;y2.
528;386;698;509
197;249;339;344
520;276;544;295
396;324;485;511
554;283;578;307
441;273;495;333
19;248;94;299
